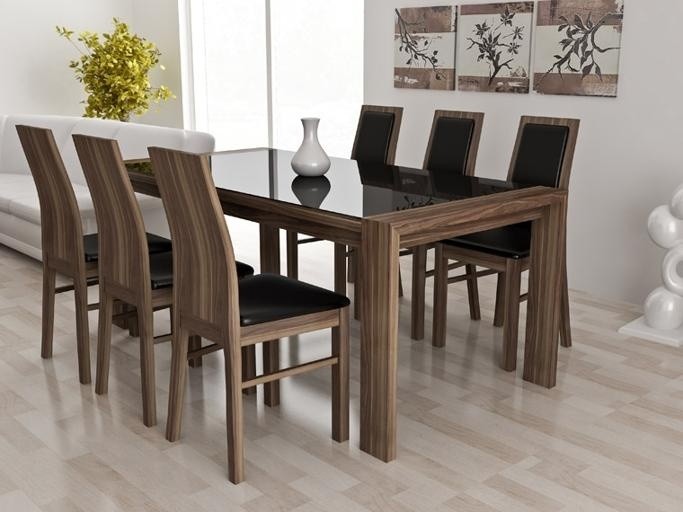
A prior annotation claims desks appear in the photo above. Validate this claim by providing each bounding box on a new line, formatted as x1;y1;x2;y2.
119;148;568;462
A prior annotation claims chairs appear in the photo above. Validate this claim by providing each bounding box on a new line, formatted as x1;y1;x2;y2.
73;135;256;427
14;124;173;384
352;109;484;340
287;106;405;319
432;116;581;372
148;147;350;485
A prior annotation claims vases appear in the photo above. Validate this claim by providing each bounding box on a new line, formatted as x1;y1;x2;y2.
292;117;331;177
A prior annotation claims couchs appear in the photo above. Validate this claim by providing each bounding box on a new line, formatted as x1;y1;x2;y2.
0;114;215;265
